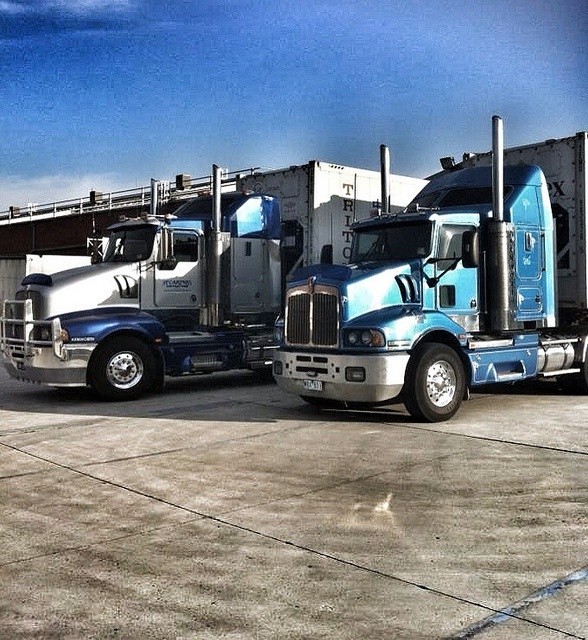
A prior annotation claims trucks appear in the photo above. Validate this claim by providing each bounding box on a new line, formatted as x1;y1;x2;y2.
0;161;432;402
270;115;588;423
